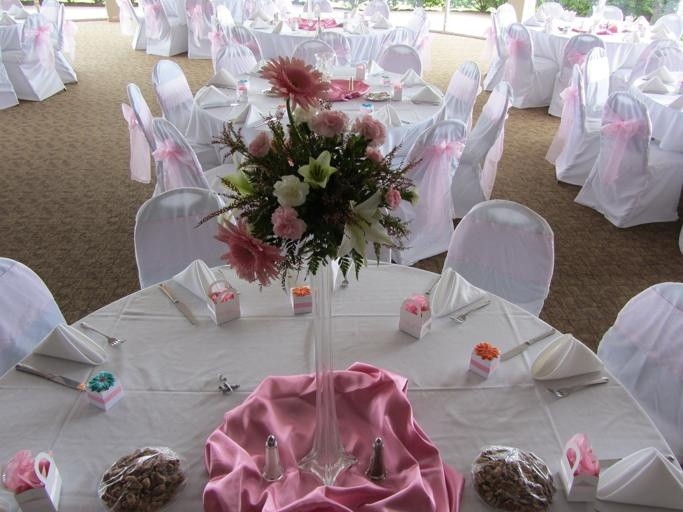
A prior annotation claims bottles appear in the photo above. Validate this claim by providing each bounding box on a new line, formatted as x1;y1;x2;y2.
260;434;283;482
364;435;388;481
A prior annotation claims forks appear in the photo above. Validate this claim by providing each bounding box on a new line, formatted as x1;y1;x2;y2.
450;302;492;325
79;320;126;348
545;376;607;398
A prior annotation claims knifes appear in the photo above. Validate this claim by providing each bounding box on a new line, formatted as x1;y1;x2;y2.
439;298;484;320
159;284;201;328
498;330;557;360
16;362;87;394
587;453;678;472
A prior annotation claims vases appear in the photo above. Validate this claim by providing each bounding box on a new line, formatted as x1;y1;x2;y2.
299;241;357;488
